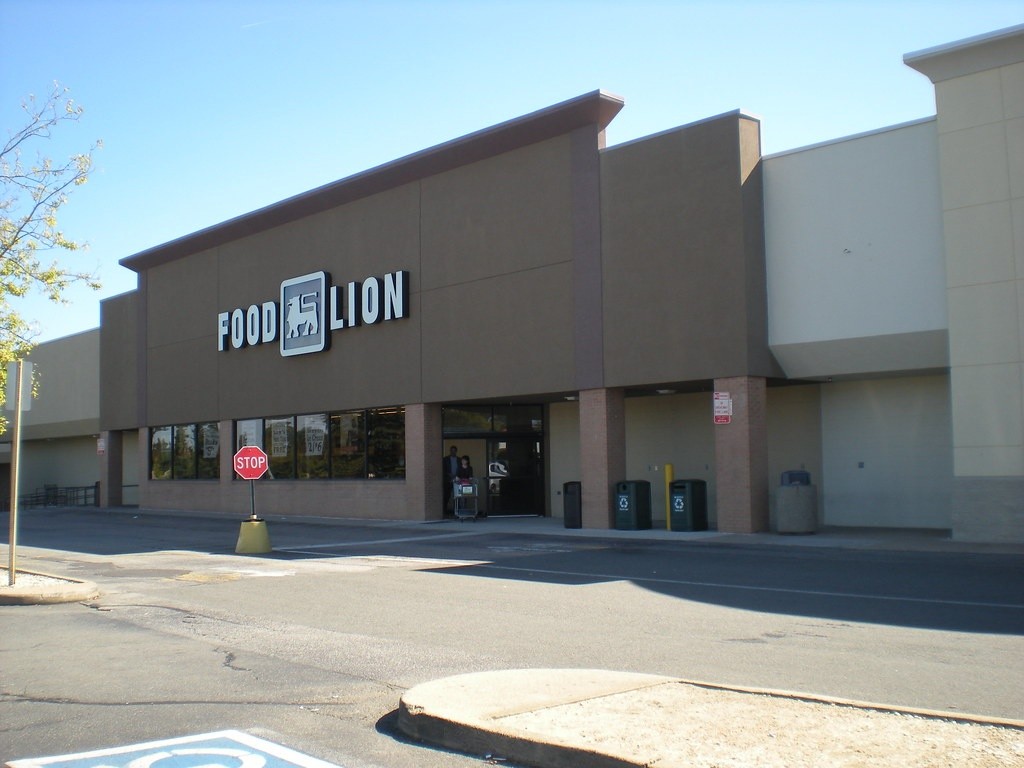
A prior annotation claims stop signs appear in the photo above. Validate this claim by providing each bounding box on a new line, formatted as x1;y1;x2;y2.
233;446;269;480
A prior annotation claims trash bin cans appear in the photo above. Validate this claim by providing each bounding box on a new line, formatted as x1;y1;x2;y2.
669;479;708;531
614;480;653;530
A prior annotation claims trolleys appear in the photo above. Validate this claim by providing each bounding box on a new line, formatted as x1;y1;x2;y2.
452;477;479;523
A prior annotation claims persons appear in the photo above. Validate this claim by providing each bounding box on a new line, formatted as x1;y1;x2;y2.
444;445;460;512
455;455;473;509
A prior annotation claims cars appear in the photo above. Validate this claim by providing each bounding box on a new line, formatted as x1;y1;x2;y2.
489;462;507;494
